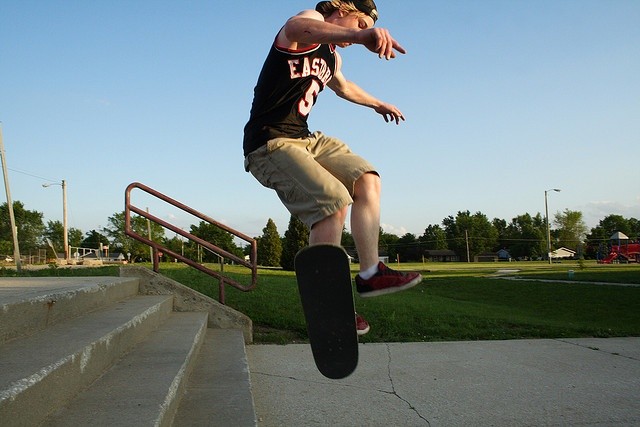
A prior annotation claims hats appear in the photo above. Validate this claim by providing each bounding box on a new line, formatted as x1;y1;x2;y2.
315;0;379;22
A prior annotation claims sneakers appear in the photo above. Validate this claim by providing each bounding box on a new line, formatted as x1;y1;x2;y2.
355;311;370;334
356;261;421;298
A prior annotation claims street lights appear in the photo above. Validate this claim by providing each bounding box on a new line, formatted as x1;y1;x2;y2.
545;188;562;264
42;179;68;261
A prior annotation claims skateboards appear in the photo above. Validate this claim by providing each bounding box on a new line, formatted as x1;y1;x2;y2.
293;241;359;379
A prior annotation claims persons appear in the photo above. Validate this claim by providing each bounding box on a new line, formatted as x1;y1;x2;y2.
242;0;424;335
